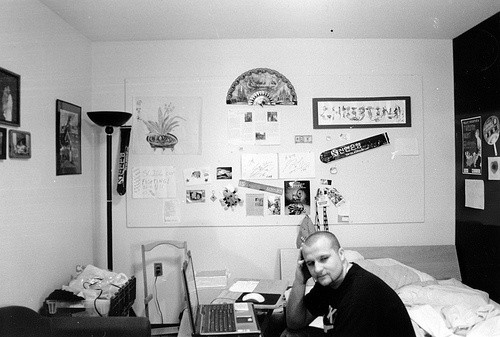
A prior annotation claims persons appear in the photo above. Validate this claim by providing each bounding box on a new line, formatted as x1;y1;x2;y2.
280;231;416;337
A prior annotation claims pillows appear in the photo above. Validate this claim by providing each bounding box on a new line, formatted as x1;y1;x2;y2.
357;257;438;290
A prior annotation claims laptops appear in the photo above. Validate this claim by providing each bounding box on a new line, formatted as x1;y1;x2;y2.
182;251;261;337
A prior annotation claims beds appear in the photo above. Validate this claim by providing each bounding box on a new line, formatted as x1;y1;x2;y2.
280;245;500;337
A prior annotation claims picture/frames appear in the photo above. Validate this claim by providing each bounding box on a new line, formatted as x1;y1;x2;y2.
56;99;82;176
0;67;21;127
0;127;6;159
9;130;31;158
313;96;411;129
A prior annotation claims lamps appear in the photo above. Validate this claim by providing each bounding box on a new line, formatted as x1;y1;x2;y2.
87;111;132;272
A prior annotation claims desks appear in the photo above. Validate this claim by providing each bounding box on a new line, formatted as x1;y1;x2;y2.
211;278;289;337
42;271;136;318
177;306;261;337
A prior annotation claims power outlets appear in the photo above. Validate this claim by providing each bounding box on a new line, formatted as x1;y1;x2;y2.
154;263;162;276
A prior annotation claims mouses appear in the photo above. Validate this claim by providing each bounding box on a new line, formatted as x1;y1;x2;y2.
242;293;266;303
